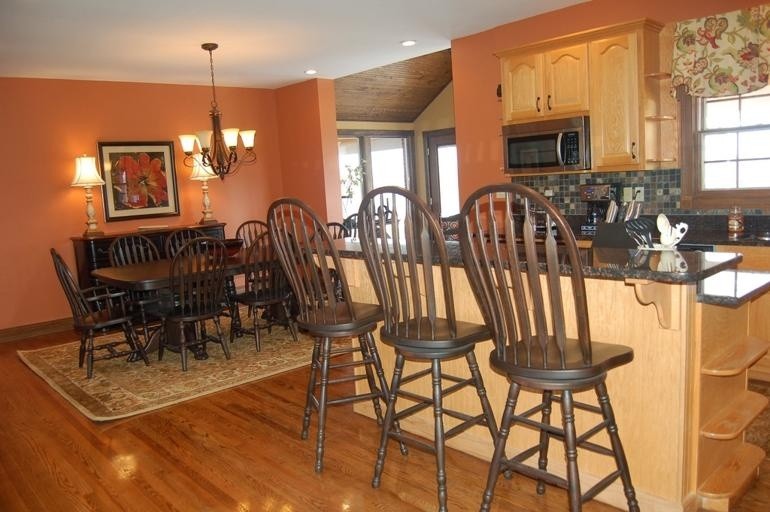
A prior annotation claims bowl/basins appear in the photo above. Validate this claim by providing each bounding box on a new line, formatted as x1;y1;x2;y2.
201;239;243;259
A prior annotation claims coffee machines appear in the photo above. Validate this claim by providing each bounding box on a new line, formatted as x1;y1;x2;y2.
579;183;620;232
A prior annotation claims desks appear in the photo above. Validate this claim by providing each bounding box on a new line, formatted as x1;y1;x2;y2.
90;241;314;354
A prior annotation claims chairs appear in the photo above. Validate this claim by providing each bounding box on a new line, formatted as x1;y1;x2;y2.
337;213;366;240
50;247;152;377
234;229;301;352
356;186;512;512
158;236;233;373
163;228;243;342
307;221;349;242
266;196;408;472
235;219;294;250
106;234;178;345
458;182;640;512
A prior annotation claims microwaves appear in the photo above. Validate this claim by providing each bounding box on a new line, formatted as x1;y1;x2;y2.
501;116;590;175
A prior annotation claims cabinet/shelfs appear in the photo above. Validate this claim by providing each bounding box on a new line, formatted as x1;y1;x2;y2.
642;18;682;171
699;254;770;512
68;222;229;327
492;31;590;126
587;17;643;172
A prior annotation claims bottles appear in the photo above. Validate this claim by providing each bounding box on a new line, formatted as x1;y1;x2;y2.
727;206;744;234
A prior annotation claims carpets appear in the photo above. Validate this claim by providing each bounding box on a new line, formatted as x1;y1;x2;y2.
18;303;356;425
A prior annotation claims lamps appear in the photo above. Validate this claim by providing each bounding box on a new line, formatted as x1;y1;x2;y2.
188;153;220;224
176;41;259;178
70;154;108;238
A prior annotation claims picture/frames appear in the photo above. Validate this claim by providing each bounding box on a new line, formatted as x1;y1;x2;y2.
96;140;180;221
